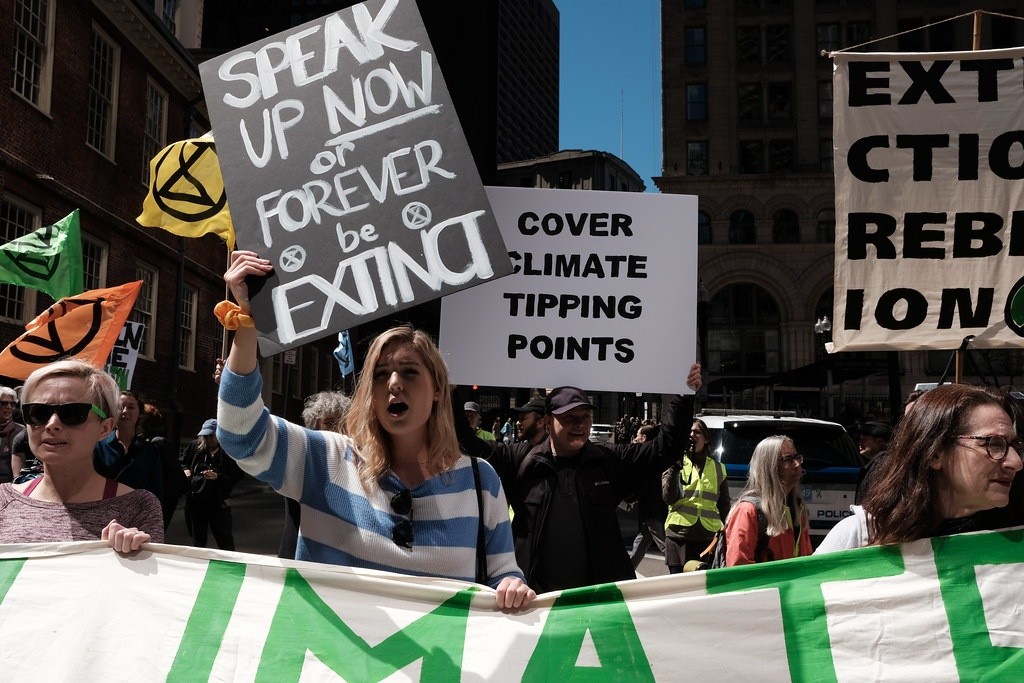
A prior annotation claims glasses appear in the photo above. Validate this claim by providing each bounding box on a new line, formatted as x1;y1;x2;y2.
0;400;17;407
950;435;1024;461
390;489;414;551
21;402;107;427
777;454;804;465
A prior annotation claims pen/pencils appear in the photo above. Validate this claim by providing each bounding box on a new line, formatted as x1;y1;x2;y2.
209;464;211;470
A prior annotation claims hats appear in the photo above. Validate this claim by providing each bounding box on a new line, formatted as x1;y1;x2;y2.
545;386;599;414
464;401;481;413
197;419;217;436
511;399;545;413
853;421;891;441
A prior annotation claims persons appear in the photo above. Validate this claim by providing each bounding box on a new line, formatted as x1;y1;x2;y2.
451;363;730;596
725;435;813;567
811;383;1024;556
217;250;535;609
177;419;237;552
0;358;192;553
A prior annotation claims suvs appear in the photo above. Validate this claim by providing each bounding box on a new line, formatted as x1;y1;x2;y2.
692;407;859;541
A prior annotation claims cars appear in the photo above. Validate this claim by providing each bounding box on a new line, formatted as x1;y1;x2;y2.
589;424;612;445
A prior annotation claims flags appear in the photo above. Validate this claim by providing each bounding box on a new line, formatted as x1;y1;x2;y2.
334;329;354;379
136;130;236;247
0;280;144;380
0;207;84;301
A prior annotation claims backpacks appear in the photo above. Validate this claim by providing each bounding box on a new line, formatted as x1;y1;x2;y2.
700;497;801;569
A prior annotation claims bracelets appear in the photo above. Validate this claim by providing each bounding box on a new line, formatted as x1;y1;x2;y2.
214;300;254;330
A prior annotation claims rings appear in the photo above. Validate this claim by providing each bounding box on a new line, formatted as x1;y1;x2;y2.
135;531;139;534
